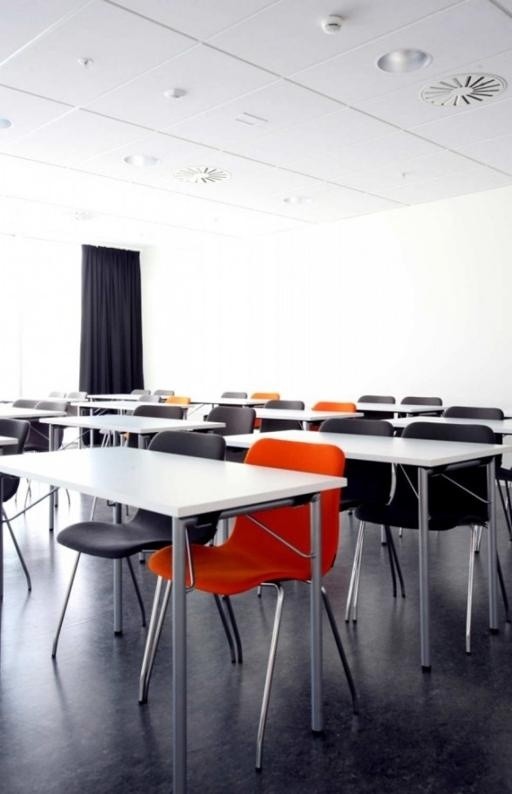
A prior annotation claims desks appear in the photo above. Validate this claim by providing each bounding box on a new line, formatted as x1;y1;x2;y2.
37;411;228;568
223;428;512;674
0;442;352;794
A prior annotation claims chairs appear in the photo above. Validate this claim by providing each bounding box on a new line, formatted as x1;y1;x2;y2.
0;417;35;609
343;419;512;655
46;428;245;707
0;388;444;534
134;438;364;771
443;405;511;551
293;416;399;626
